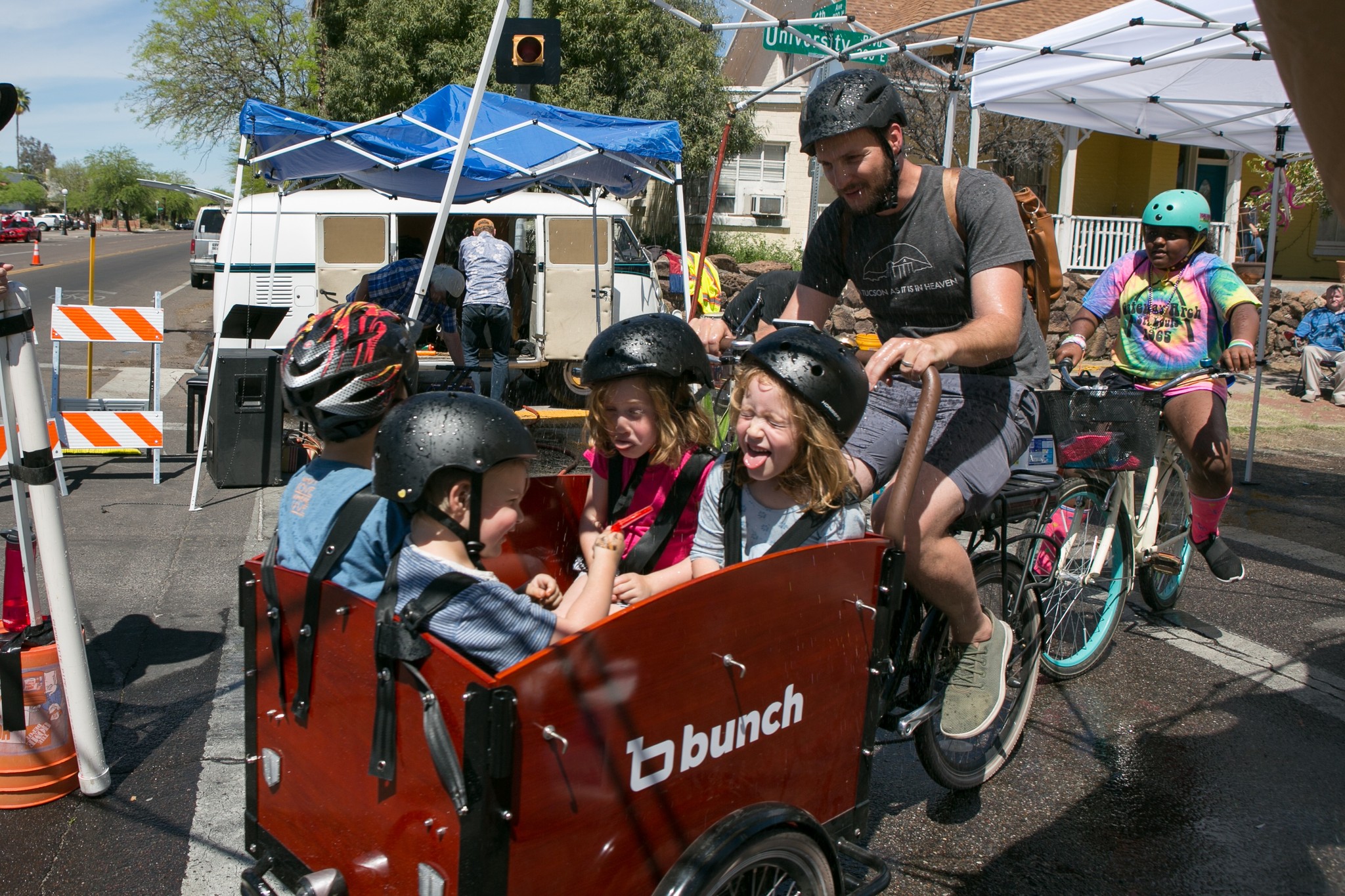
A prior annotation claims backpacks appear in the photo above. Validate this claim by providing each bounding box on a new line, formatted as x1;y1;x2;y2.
842;168;1064;343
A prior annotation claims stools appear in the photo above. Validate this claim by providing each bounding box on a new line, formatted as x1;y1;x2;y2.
186;373;208;453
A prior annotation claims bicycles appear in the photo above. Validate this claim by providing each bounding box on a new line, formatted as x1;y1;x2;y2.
1024;353;1257;680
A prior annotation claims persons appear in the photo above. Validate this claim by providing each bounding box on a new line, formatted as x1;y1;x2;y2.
1240;186;1265;262
275;303;419;601
1030;190;1263;599
720;270;804;339
371;392;626;676
575;312;723;615
689;326;869;580
688;69;1056;739
346;258;475;415
459;218;514;404
1295;285;1345;406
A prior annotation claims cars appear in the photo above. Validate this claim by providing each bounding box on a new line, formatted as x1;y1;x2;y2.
66;213;81;231
175;218;195;231
0;221;42;243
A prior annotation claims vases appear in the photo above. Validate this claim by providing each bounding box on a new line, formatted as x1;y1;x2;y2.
1336;260;1345;283
1232;261;1266;285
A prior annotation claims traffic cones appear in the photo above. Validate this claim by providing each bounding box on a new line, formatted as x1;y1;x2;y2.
29;240;44;266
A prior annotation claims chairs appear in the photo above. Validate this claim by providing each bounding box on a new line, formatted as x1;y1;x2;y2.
1284;331;1336;396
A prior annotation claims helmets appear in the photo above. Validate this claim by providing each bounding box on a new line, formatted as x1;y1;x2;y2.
580;313;716;392
799;68;907;157
739;326;868;446
277;302;418;443
371;390;539;504
1141;190;1210;238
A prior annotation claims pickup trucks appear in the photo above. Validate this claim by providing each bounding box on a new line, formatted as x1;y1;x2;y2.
11;210;60;232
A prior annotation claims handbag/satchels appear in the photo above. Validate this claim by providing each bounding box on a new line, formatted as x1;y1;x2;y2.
1099;367;1144;389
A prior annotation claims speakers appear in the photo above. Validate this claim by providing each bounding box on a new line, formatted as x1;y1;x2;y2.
209;346;285;485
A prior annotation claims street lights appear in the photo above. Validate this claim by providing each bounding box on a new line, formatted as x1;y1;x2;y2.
61;188;68;236
155;200;159;225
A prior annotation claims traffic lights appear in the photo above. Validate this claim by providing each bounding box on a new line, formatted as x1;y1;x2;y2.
512;34;544;66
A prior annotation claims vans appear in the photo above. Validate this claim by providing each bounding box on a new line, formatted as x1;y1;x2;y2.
211;186;665;409
189;205;232;288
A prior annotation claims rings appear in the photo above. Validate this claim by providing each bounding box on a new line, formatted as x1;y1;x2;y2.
901;360;914;368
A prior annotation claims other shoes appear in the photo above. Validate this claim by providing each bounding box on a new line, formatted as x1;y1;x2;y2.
1330;397;1345;406
1301;394;1320;402
1187;513;1245;584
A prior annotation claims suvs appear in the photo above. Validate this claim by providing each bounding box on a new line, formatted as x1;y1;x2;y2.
0;213;30;229
37;213;67;231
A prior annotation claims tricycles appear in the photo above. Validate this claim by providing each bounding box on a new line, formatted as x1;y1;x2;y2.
235;334;1068;896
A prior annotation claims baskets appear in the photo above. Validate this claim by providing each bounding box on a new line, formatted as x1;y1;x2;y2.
1041;389;1163;471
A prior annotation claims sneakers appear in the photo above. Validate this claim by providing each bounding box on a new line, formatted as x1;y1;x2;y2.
939;603;1013;740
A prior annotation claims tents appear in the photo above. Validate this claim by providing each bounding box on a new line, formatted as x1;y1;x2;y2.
188;85;692;512
969;0;1316;480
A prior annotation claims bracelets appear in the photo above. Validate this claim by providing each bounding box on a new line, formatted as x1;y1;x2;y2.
1228;339;1254;350
1061;334;1086;351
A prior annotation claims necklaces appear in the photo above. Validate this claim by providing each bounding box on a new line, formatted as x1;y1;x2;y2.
1148;262;1185;330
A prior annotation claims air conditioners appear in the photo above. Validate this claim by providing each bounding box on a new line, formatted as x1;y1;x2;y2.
751;195;784;216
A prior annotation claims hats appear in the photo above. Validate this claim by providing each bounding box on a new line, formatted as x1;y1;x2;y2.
474;218;494;231
442;268;465;309
